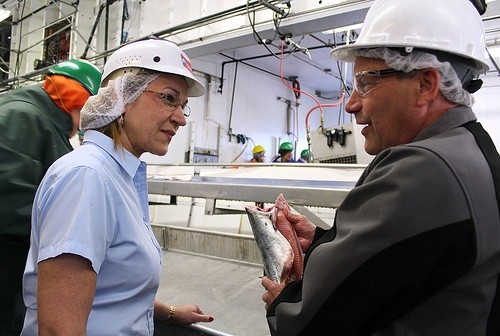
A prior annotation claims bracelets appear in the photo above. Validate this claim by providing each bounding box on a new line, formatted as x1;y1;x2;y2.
166;305;177;323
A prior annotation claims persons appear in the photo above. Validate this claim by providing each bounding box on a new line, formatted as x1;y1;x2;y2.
19;39;215;336
0;58;102;336
261;0;500;336
251;142;309;163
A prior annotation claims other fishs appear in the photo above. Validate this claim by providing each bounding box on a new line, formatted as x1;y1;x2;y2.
244;193;305;286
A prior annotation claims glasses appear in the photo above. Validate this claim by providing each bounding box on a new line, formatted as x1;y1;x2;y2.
354;68;423;97
143;90;191;117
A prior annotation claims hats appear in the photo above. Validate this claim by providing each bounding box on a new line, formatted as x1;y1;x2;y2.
301;150;313;158
279;142;293;153
330;0;490;72
101;40;206;98
47;58;101;96
253;145;264;154
